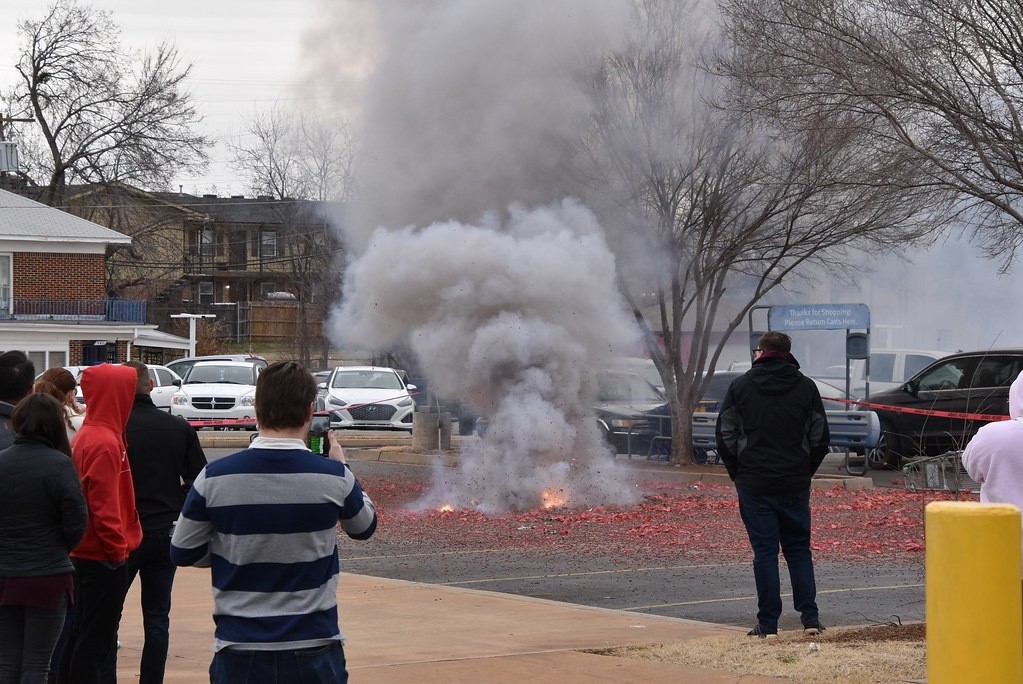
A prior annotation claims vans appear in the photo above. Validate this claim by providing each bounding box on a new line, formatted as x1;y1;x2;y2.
861;348;965;399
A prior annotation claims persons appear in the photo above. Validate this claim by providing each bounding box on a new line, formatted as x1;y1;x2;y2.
168;359;378;683
959;367;1023;519
0;391;89;684
34;366;90;684
67;361;145;684
714;331;832;640
115;360;209;684
1;350;38;452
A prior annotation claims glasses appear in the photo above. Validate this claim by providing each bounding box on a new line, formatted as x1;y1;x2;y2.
753;348;762;354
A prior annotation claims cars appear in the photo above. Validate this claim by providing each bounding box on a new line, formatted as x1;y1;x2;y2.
317;364;419;435
854;348;1023;469
164;355;262;381
307;364;453;410
678;338;861;467
458;394;491;439
170;361;268;431
594;370;671;461
32;361;182;416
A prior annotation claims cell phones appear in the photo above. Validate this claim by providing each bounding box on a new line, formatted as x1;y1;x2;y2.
306;414;331;457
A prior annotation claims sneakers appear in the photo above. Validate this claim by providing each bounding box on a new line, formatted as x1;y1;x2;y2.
805;620;826;635
746;625;780;641
